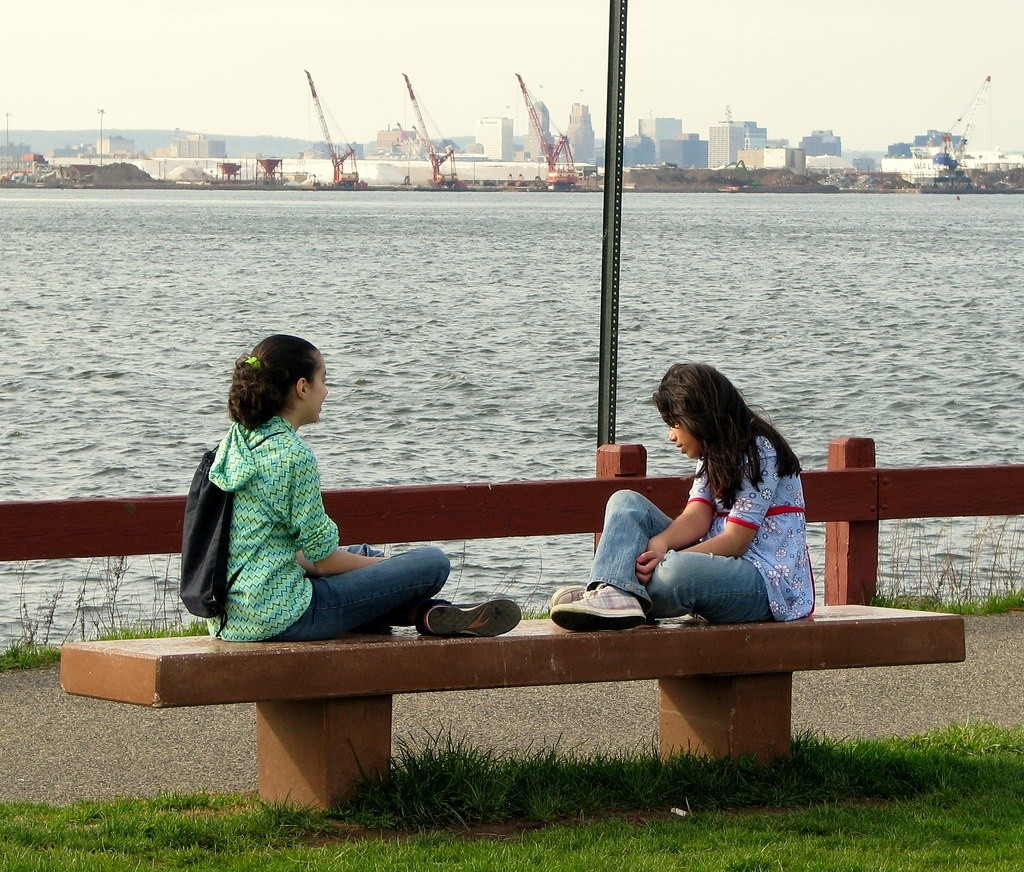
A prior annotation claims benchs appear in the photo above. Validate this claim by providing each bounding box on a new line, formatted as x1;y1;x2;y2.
60;604;967;812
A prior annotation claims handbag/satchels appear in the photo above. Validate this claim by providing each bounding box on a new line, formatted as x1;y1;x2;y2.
178;451;233;619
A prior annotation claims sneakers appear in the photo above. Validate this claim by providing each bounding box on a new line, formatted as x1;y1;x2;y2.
549;583;646;632
551;586;589;606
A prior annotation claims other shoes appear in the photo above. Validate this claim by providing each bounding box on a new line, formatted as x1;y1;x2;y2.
423;599;522;637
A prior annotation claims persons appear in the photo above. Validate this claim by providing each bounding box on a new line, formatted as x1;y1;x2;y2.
207;335;522;637
551;363;815;633
